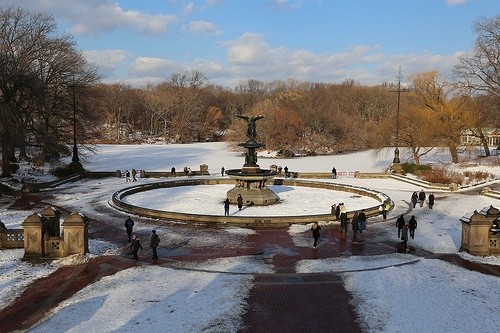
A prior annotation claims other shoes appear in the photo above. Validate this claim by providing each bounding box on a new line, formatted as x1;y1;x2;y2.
313;246;318;249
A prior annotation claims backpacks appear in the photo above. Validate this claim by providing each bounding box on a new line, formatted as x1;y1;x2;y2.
128;221;132;226
155;237;160;244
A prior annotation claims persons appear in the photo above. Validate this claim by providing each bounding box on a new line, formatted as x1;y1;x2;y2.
220;166;225;177
149;230;160;260
224;198;230;217
237;194;243;210
125;215;134;243
130;236;143;261
171;167;175;175
332;167;336;179
429;193;434;209
311;222;321;250
412;189;425;208
278;166;282;174
331;202;369;236
125;170;131;182
184;166;189;174
396;213;417;254
381;201;391;219
284;166;288;176
132;169;137;182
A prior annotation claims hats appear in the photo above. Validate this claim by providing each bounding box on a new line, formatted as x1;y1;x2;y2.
132;234;136;239
